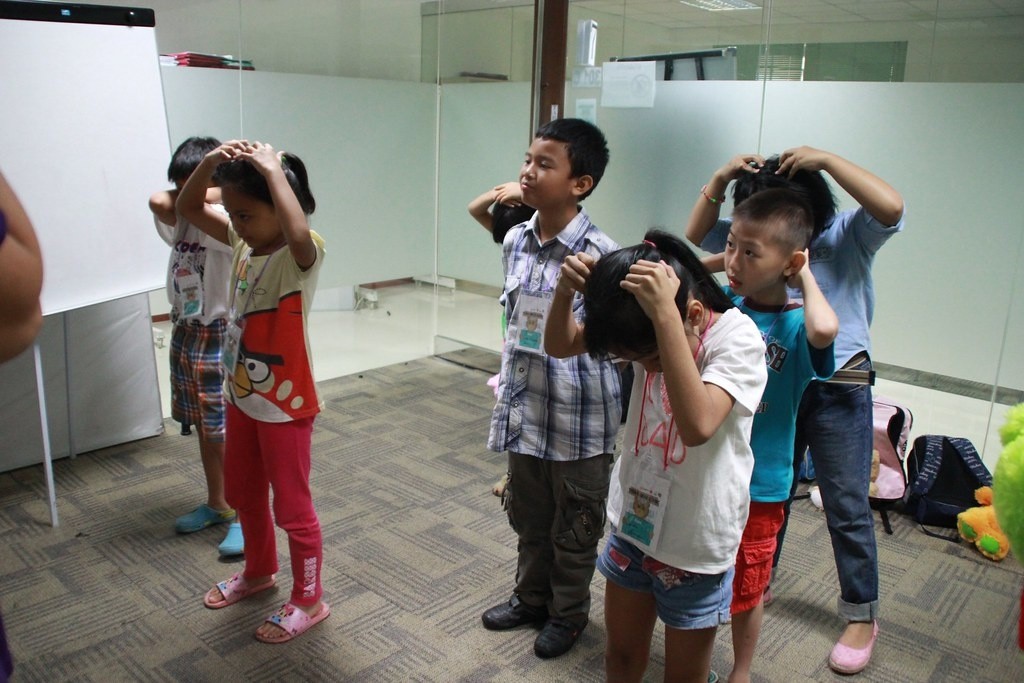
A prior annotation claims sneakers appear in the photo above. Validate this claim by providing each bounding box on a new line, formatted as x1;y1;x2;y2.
534;613;589;658
482;592;550;630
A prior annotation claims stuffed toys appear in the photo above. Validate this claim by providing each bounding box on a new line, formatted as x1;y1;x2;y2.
956;403;1024;651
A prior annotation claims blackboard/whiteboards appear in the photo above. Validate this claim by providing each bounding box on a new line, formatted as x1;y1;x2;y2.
0;19;178;316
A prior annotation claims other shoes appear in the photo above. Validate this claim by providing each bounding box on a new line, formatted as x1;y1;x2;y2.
829;616;879;672
724;589;771;624
492;473;510;496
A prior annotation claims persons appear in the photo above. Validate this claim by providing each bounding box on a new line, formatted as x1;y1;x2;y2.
544;226;769;683
700;189;839;683
150;136;251;556
175;140;331;642
0;169;45;681
685;145;905;674
469;118;624;657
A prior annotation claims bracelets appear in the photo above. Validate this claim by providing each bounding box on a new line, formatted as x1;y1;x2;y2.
702;185;726;203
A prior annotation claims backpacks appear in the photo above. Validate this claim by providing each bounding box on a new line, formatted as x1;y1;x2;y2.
869;393;914;533
907;432;995;542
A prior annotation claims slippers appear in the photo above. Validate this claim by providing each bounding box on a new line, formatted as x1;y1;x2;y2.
217;522;245;556
174;503;236;533
256;596;330;642
204;571;277;609
